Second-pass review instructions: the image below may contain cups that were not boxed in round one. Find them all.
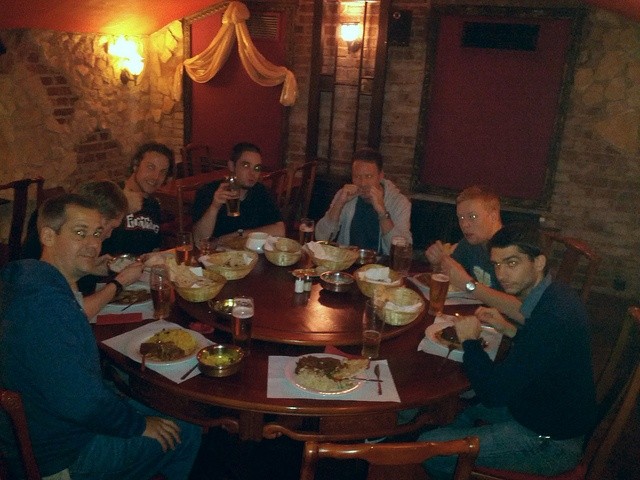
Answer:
[200,237,217,257]
[362,300,385,333]
[362,330,381,359]
[298,218,314,248]
[428,274,455,313]
[149,263,168,314]
[176,230,193,266]
[233,295,253,339]
[223,174,242,217]
[391,235,406,271]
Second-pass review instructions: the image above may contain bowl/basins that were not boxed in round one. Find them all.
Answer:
[245,233,270,253]
[208,298,233,323]
[106,254,141,277]
[355,248,377,265]
[320,270,354,293]
[198,344,245,379]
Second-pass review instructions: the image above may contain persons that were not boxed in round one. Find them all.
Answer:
[100,141,175,257]
[316,151,413,255]
[419,221,599,476]
[191,141,286,245]
[425,185,532,324]
[17,179,144,321]
[0,194,203,479]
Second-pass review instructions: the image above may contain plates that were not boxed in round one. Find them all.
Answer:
[89,280,157,307]
[285,353,366,394]
[128,327,202,366]
[293,268,321,278]
[413,271,466,292]
[425,322,496,352]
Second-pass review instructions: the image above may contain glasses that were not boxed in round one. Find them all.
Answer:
[235,160,263,170]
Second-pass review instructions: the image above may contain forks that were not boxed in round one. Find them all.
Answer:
[437,344,459,372]
[374,363,383,396]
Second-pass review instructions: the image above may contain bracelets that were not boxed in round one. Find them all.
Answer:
[109,280,124,295]
[238,228,244,234]
[379,212,390,219]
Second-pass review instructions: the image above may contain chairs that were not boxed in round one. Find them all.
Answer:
[180,143,213,178]
[0,175,45,261]
[0,392,41,480]
[473,305,640,480]
[290,161,319,225]
[300,436,481,479]
[537,230,602,304]
[178,182,202,232]
[259,170,289,220]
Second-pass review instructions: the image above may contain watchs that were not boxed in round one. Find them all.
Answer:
[465,279,478,293]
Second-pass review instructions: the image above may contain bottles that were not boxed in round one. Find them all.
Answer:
[294,275,304,294]
[304,274,312,291]
